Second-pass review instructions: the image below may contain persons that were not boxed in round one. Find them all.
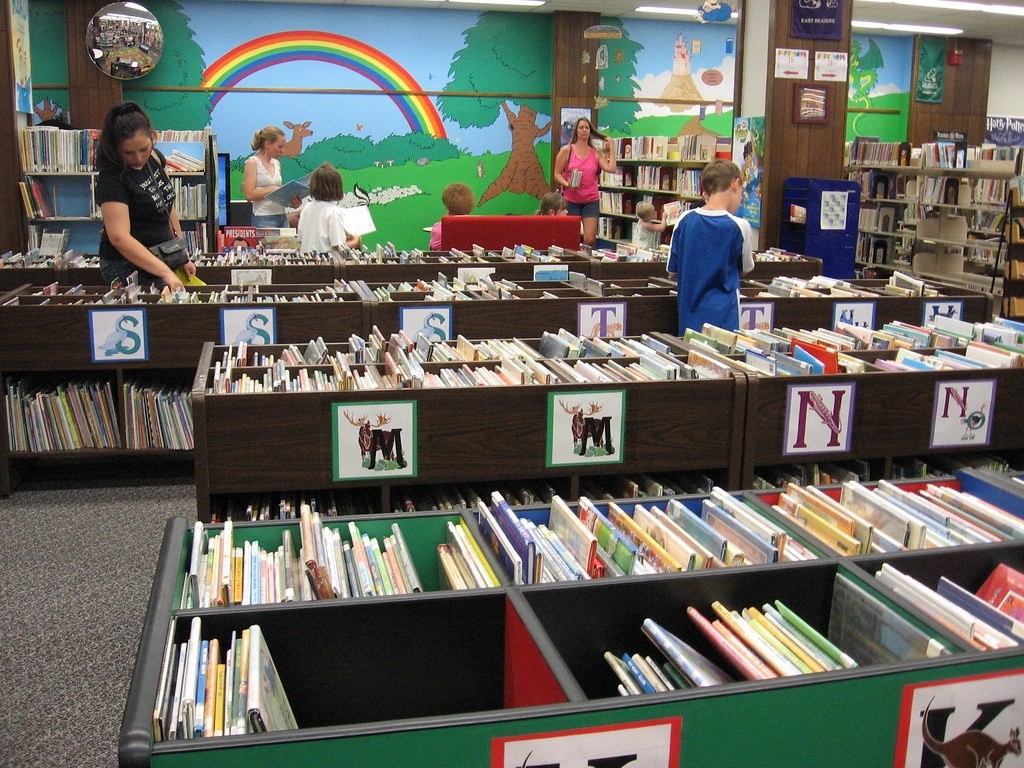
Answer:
[244,125,302,228]
[298,164,360,253]
[429,182,476,250]
[636,201,668,252]
[535,117,616,249]
[666,161,754,336]
[95,102,196,293]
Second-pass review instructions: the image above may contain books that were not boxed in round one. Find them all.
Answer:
[153,453,1024,742]
[0,126,1024,452]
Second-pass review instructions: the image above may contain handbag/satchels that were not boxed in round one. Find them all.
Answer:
[148,238,190,270]
[555,186,564,197]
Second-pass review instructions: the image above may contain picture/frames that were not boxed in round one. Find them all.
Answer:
[793,81,832,127]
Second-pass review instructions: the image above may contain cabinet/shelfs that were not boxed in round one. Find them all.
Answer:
[15,142,216,255]
[0,246,1024,768]
[839,167,1024,319]
[594,157,708,252]
